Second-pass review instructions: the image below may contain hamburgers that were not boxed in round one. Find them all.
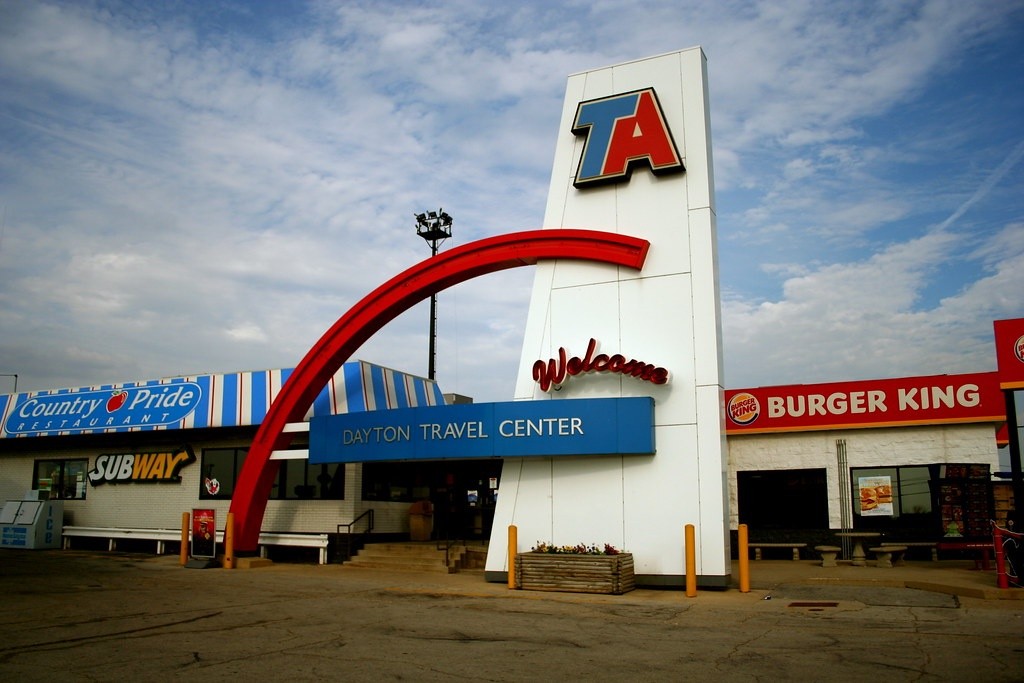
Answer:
[875,485,892,502]
[860,488,878,510]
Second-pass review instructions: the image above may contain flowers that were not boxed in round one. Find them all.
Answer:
[532,541,620,555]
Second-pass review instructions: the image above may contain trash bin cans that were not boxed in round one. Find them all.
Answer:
[409,500,432,541]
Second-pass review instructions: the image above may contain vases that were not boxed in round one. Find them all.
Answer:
[515,552,636,595]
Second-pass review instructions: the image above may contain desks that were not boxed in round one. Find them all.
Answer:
[835,532,881,566]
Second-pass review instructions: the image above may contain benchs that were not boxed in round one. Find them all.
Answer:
[870,546,910,568]
[881,543,938,563]
[748,543,807,561]
[815,545,842,567]
[937,543,995,570]
